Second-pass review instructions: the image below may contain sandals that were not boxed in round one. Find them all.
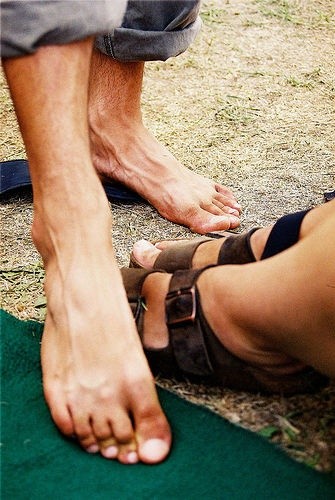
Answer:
[129,207,309,274]
[114,268,329,392]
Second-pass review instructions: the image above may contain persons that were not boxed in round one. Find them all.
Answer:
[1,0,242,464]
[120,192,334,397]
